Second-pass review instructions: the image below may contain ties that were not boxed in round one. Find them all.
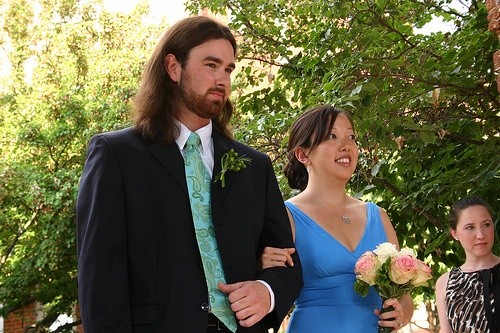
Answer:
[183,132,240,333]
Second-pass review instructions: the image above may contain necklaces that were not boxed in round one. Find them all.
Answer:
[299,191,351,223]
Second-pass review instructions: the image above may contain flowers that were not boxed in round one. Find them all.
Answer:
[354,242,434,333]
[215,148,253,188]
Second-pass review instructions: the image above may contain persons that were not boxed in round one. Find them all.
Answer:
[76,15,303,333]
[435,196,500,333]
[261,103,414,333]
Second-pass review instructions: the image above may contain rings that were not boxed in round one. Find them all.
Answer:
[398,319,402,327]
